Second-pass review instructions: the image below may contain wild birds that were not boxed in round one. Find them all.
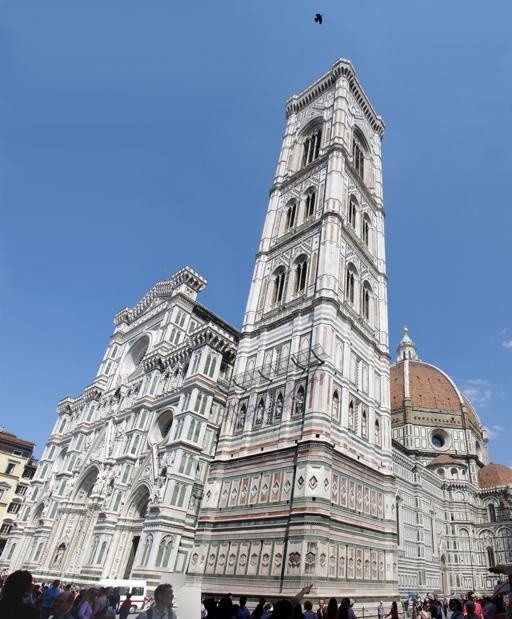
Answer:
[314,14,323,24]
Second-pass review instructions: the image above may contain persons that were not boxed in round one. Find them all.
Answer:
[384,601,398,619]
[201,584,357,619]
[0,568,132,619]
[135,583,177,619]
[402,580,512,619]
[377,600,384,619]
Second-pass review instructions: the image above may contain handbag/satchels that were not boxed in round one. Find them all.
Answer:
[71,600,88,617]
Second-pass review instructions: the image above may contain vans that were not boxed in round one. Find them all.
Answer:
[96,579,147,614]
